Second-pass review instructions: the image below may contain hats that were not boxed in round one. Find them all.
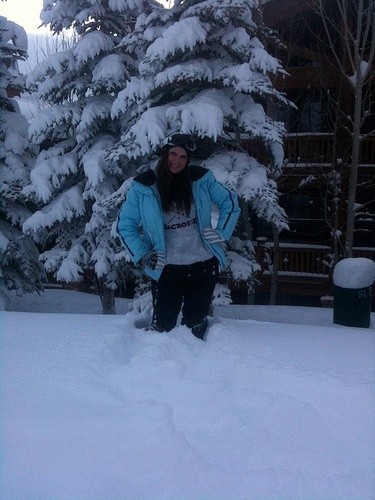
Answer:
[155,134,193,164]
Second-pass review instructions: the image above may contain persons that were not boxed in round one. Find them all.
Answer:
[115,134,240,341]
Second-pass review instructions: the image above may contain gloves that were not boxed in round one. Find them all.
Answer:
[138,251,157,270]
[202,225,225,243]
